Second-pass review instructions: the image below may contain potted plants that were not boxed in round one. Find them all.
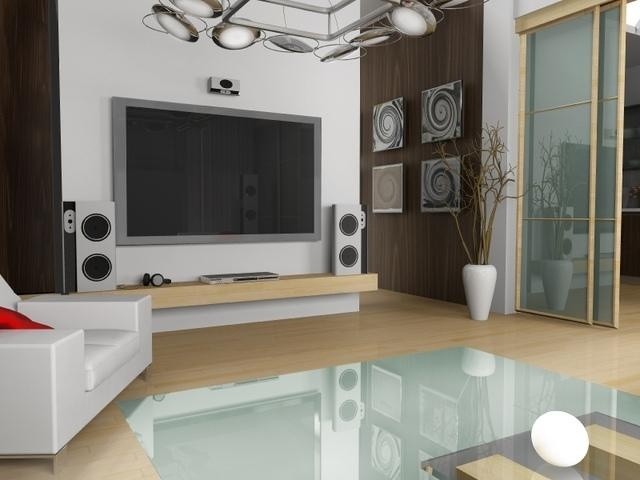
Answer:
[530,127,589,311]
[445,347,497,456]
[430,120,530,321]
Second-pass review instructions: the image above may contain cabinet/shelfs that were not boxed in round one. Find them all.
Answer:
[68,272,378,308]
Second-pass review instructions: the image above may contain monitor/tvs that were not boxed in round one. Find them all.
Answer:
[110,96,322,246]
[560,140,616,235]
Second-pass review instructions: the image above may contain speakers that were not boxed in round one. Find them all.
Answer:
[241,173,260,234]
[548,205,576,264]
[331,203,368,276]
[61,200,117,294]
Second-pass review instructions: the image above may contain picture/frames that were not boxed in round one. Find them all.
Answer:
[420,156,461,213]
[371,96,406,153]
[372,162,404,214]
[421,79,464,144]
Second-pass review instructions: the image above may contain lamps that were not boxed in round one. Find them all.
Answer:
[142,3,207,42]
[158,0,231,18]
[206,17,266,50]
[263,32,319,57]
[377,1,444,39]
[314,44,367,62]
[343,25,402,46]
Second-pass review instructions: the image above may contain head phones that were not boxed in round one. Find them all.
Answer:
[142,273,172,287]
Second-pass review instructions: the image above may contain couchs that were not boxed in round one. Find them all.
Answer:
[0,276,151,474]
